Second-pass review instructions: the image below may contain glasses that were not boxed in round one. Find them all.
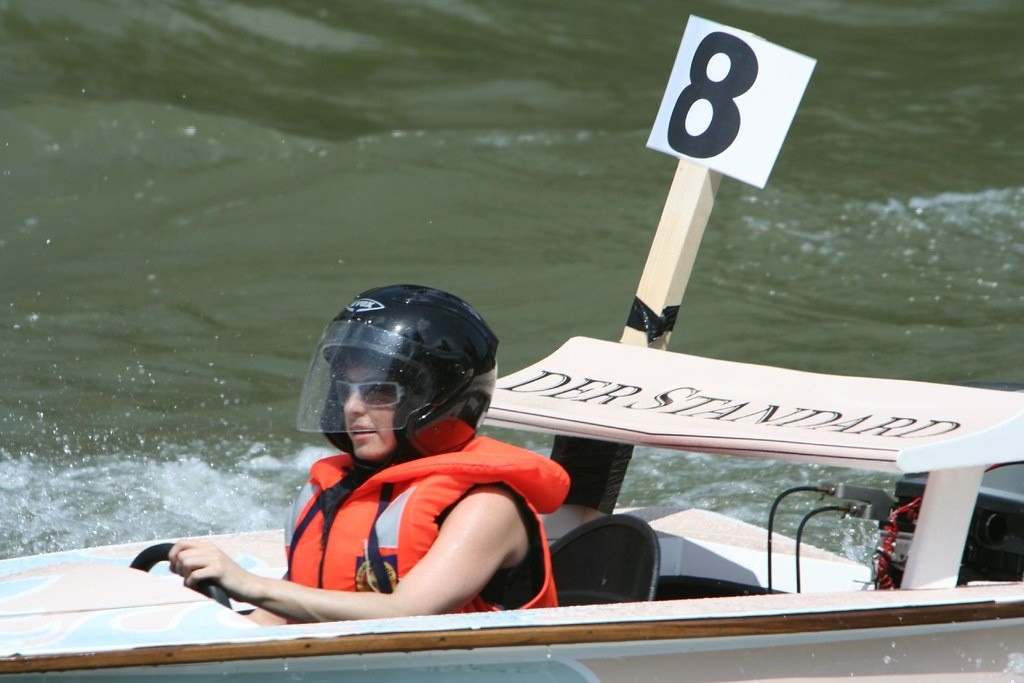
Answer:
[336,381,406,407]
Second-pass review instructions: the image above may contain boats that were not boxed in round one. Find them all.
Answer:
[0,15,1024,683]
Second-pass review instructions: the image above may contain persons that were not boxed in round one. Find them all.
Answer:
[169,285,571,627]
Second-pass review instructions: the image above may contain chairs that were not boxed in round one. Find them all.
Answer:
[549,513,661,607]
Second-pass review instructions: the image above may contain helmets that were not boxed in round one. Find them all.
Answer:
[296,284,499,453]
